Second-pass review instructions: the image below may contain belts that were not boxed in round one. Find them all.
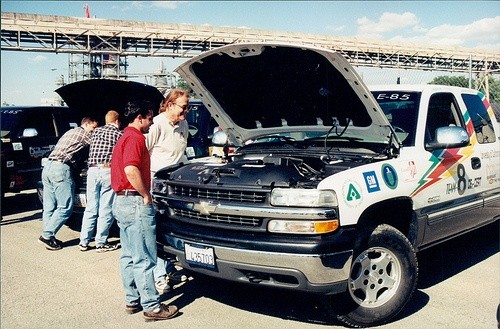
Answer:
[49,158,70,164]
[89,164,112,168]
[116,191,141,196]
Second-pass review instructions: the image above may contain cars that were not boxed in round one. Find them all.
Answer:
[37,77,219,229]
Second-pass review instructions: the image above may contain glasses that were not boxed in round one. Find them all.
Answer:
[173,103,193,112]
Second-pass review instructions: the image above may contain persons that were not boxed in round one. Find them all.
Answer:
[111,97,180,321]
[38,114,99,250]
[146,86,190,294]
[79,109,122,253]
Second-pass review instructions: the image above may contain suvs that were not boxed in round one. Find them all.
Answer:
[1,105,76,195]
[148,39,499,329]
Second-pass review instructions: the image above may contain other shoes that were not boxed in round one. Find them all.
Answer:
[96,244,116,252]
[142,304,179,321]
[154,281,172,294]
[78,244,89,251]
[38,236,62,251]
[166,270,183,283]
[125,305,143,315]
